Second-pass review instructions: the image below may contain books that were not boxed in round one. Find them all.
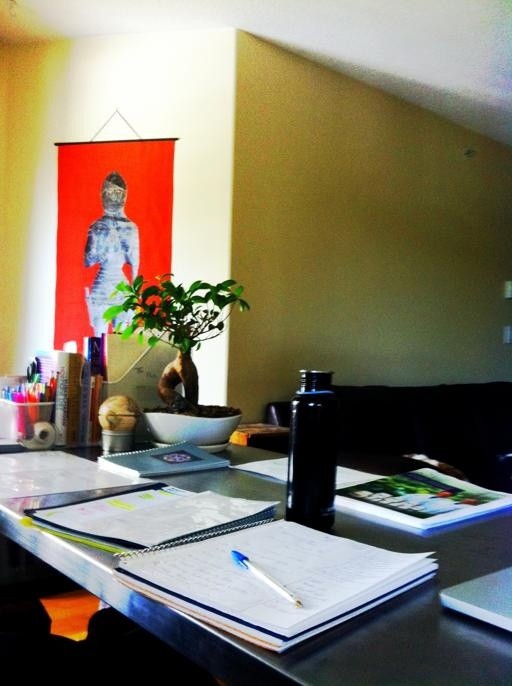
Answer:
[102,331,158,382]
[85,336,107,378]
[55,352,85,446]
[333,466,512,530]
[96,441,233,475]
[112,516,439,653]
[20,481,281,552]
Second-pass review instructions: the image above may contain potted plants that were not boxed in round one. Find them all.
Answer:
[101,271,253,454]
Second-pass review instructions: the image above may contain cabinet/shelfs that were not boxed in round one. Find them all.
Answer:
[228,423,290,455]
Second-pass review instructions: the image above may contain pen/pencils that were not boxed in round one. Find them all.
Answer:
[231,547,310,612]
[49,368,59,383]
[34,374,42,383]
[3,383,61,437]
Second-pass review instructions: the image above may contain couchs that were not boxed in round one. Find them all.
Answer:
[263,380,512,494]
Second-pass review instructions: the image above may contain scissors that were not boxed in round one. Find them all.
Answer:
[26,356,41,383]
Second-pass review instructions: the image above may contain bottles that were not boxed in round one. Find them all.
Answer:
[285,370,340,531]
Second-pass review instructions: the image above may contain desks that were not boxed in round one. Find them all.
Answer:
[0,440,511,686]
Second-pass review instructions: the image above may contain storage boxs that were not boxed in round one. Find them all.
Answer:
[0,396,56,439]
